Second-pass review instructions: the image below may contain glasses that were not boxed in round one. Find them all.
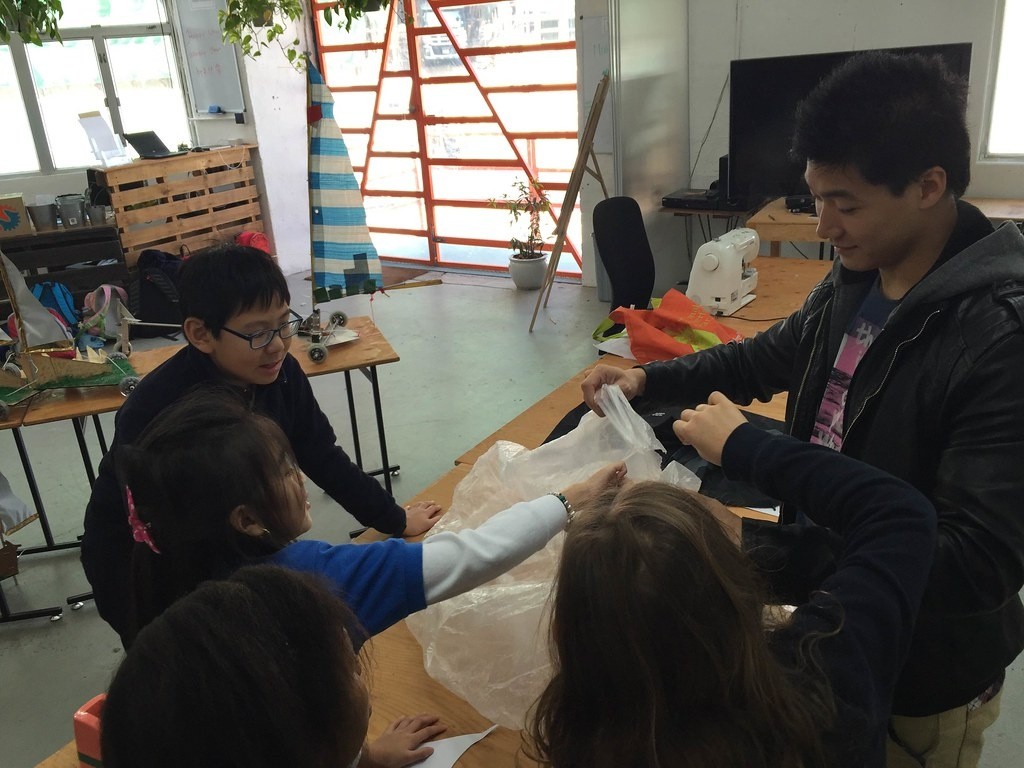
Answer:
[221,308,304,350]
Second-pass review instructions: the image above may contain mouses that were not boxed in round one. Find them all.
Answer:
[191,147,203,152]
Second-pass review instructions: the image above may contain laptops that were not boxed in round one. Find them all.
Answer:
[123,131,188,159]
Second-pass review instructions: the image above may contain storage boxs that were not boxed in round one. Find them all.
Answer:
[0,191,32,237]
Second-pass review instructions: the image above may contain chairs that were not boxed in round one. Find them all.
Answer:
[592,196,655,355]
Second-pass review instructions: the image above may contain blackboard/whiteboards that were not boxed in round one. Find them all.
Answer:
[170,0,247,114]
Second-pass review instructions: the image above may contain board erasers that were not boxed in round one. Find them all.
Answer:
[209,106,221,113]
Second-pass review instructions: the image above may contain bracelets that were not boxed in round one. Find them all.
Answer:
[546,491,574,532]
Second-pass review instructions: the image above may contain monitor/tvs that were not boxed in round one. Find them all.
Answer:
[728,43,973,203]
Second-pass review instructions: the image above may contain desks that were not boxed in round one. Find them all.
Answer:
[453,251,836,524]
[745,197,1024,260]
[0,395,64,622]
[35,462,549,768]
[22,314,401,611]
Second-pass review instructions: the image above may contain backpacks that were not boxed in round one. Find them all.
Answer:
[74,283,131,346]
[131,248,186,337]
[7,306,76,360]
[234,229,276,256]
[29,279,81,347]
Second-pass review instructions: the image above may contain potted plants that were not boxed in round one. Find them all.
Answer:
[485,176,551,291]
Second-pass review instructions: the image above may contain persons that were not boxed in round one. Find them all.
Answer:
[98,568,445,768]
[581,53,1024,768]
[113,398,628,655]
[81,243,441,658]
[521,390,939,768]
